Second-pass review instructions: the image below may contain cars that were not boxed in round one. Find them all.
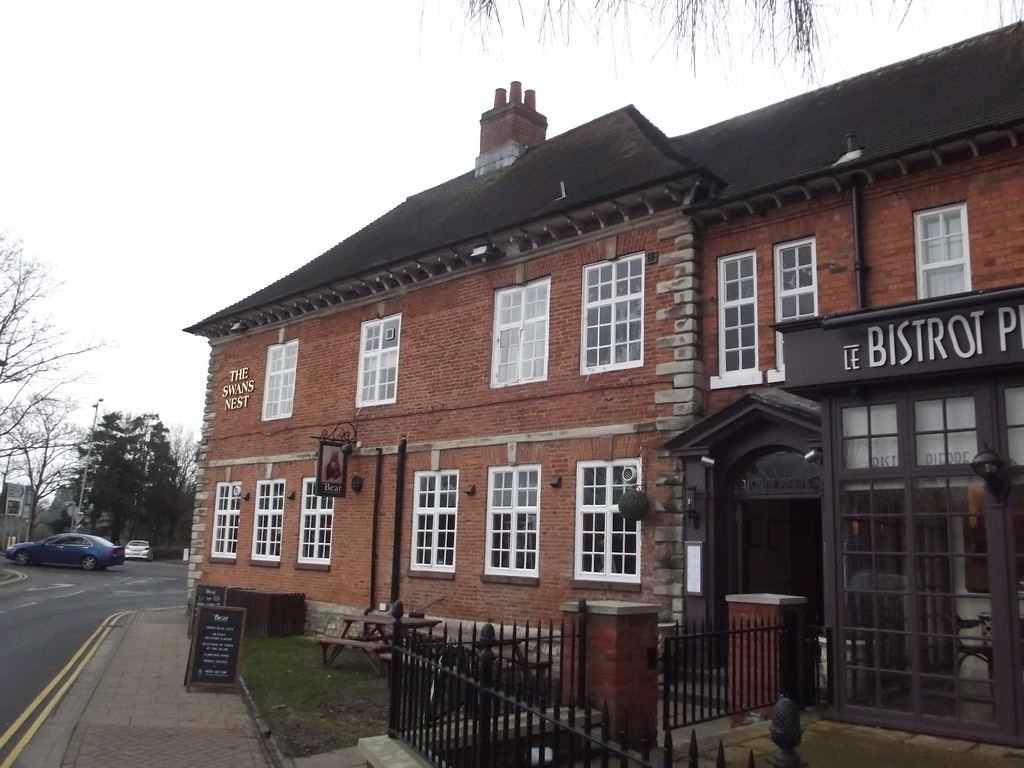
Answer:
[5,532,126,571]
[124,539,153,562]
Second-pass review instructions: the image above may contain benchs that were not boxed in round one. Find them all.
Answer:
[384,630,443,642]
[317,637,392,676]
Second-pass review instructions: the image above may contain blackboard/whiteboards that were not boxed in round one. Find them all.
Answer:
[187,605,247,685]
[180,584,228,638]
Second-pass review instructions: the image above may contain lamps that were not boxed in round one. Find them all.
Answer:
[684,484,699,531]
[967,442,1008,504]
[804,450,821,470]
[699,455,716,468]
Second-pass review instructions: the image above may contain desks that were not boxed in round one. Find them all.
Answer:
[327,615,443,676]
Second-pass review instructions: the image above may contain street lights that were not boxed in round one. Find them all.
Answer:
[74,398,105,530]
